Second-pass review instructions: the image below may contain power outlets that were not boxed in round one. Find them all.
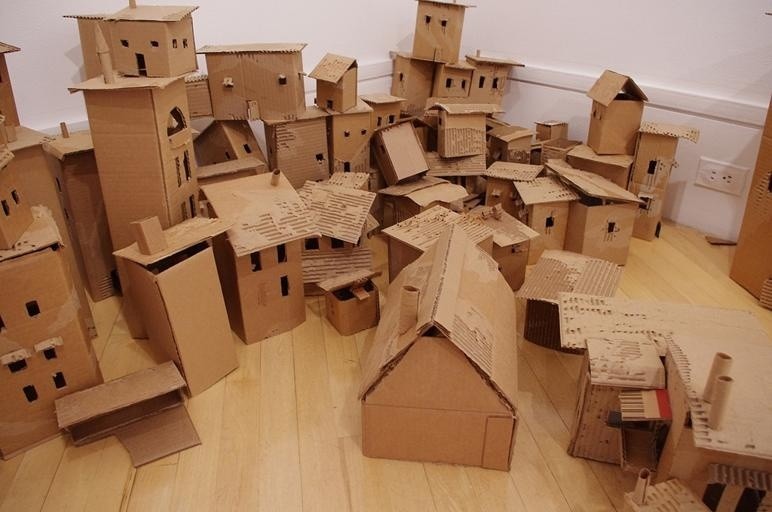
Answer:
[691,157,751,196]
